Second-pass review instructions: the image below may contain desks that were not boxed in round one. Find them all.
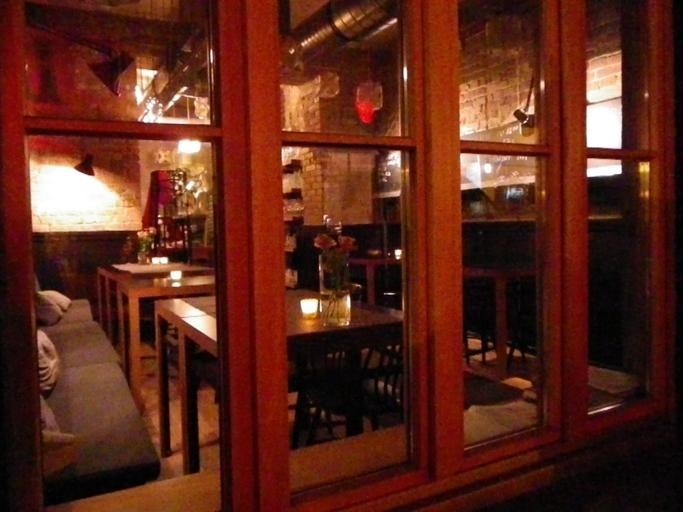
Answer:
[96,261,216,350]
[154,290,403,475]
[114,276,215,417]
[318,255,401,304]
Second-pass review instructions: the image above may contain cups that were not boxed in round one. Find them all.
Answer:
[152,257,168,265]
[299,297,319,320]
[171,270,182,282]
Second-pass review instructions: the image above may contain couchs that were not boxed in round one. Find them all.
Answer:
[33,274,161,483]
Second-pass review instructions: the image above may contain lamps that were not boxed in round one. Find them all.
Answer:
[74,154,96,176]
[513,78,533,124]
[27,21,136,97]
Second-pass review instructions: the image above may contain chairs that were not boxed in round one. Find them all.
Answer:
[294,336,404,448]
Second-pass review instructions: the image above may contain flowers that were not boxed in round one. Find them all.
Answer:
[309,234,363,326]
[136,227,157,251]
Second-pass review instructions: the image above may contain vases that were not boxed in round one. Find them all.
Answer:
[137,253,150,264]
[327,291,352,325]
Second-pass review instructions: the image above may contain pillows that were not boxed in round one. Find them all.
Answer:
[36,288,76,483]
